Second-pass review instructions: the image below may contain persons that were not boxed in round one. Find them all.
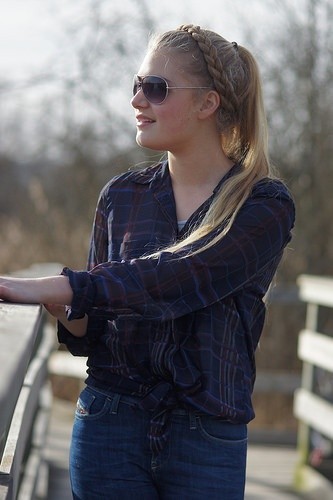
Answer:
[0,23,296,500]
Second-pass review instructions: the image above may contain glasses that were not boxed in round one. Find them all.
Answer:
[132,74,215,105]
